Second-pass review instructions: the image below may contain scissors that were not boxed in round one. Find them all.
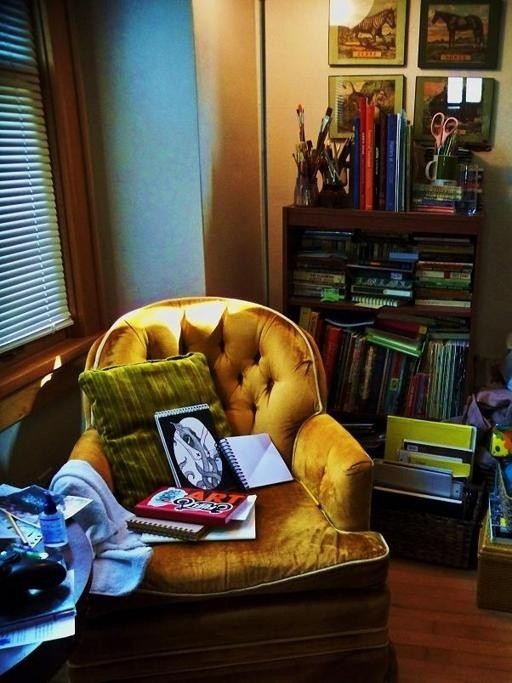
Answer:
[430,112,458,155]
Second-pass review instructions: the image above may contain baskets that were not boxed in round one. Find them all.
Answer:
[369,478,486,567]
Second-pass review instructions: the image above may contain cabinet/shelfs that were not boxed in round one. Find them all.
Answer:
[281,205,487,452]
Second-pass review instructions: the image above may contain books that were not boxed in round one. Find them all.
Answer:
[1,400,295,652]
[292,227,475,308]
[371,415,477,520]
[350,96,485,215]
[298,307,471,449]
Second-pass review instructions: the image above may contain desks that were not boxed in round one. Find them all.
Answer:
[0,518,94,683]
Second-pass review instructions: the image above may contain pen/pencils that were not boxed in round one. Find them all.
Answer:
[434,128,459,157]
[8,514,28,546]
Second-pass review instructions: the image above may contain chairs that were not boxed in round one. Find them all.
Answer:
[67,295,390,683]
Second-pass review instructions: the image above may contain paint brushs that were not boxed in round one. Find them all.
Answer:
[292,104,354,205]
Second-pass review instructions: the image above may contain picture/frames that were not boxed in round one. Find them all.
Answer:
[328,0,504,152]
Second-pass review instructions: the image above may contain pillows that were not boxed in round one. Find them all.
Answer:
[79,351,236,511]
